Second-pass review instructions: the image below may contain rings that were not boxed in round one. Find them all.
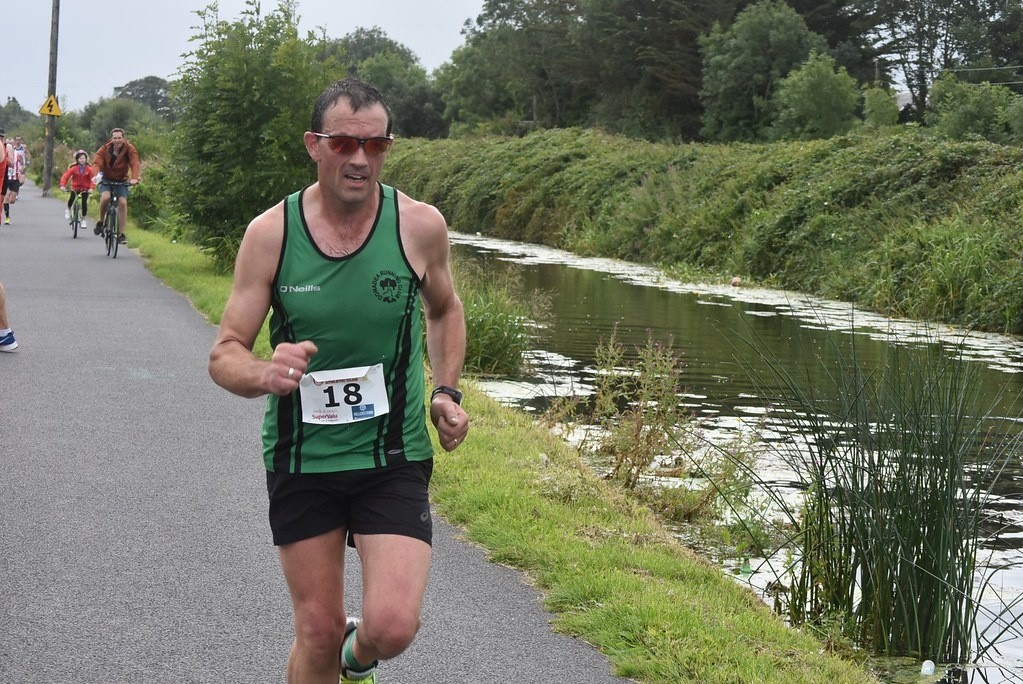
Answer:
[455,439,457,445]
[288,368,294,378]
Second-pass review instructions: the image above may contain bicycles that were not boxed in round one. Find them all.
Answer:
[61,189,92,239]
[97,180,140,260]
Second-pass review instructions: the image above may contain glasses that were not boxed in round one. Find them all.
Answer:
[314,133,394,156]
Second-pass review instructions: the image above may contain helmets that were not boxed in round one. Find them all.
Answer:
[74,150,88,159]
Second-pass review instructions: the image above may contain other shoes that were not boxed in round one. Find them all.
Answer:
[118,233,128,244]
[65,209,71,218]
[94,221,103,235]
[4,217,10,224]
[81,220,87,228]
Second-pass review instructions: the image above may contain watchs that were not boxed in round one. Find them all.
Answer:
[430,386,462,405]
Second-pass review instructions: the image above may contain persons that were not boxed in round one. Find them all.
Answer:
[60,150,95,229]
[0,282,18,351]
[92,128,139,245]
[0,129,31,226]
[209,78,469,684]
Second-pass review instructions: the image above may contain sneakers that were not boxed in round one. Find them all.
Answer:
[340,617,378,684]
[0,331,18,352]
